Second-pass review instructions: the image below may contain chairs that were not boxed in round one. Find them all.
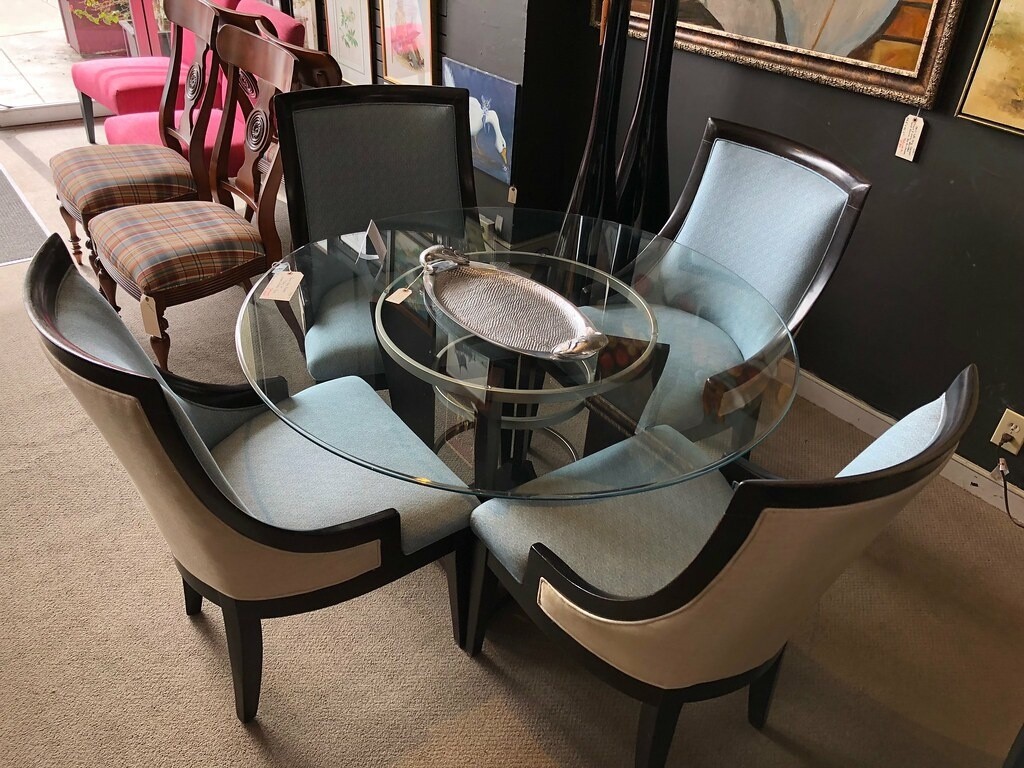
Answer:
[47,0,980,768]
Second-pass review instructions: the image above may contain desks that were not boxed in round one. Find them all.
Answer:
[234,206,797,502]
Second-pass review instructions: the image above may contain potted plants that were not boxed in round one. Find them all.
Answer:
[69,0,172,56]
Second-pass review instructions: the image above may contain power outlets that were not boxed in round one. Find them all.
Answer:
[991,407,1024,455]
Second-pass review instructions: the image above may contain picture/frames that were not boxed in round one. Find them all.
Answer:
[379,0,436,86]
[595,1,963,106]
[289,0,320,51]
[957,0,1024,138]
[324,0,375,85]
